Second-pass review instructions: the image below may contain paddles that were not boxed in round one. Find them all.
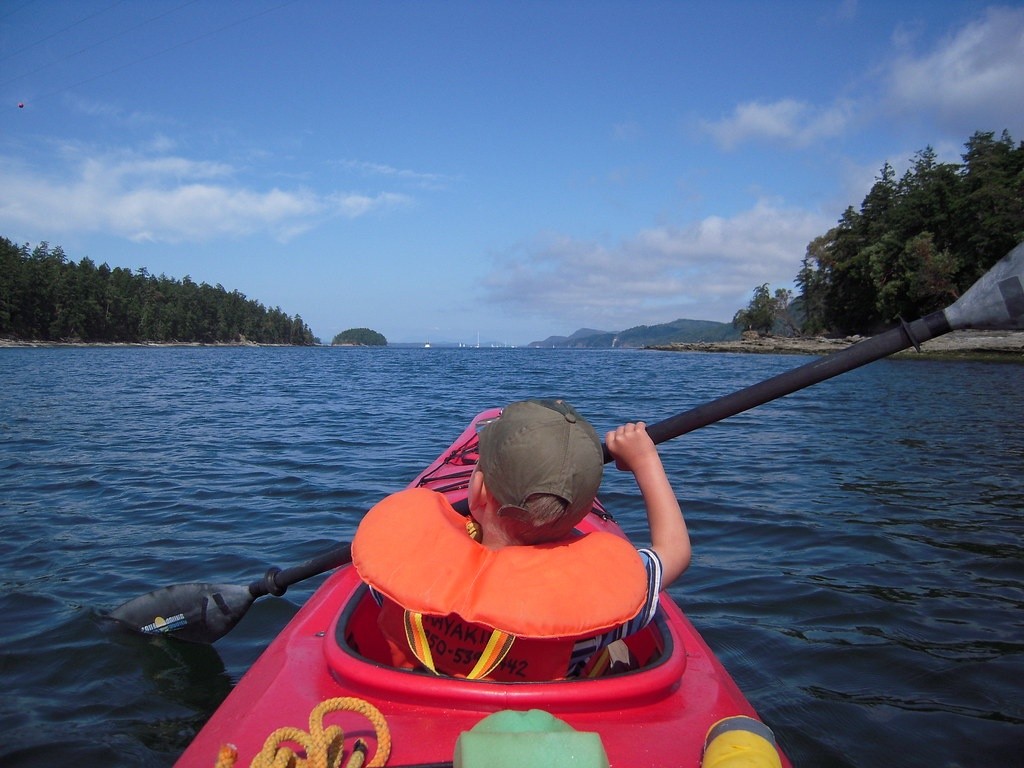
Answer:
[109,239,1024,644]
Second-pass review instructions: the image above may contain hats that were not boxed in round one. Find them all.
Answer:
[475,400,604,530]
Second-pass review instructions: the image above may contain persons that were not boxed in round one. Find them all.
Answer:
[351,396,691,688]
